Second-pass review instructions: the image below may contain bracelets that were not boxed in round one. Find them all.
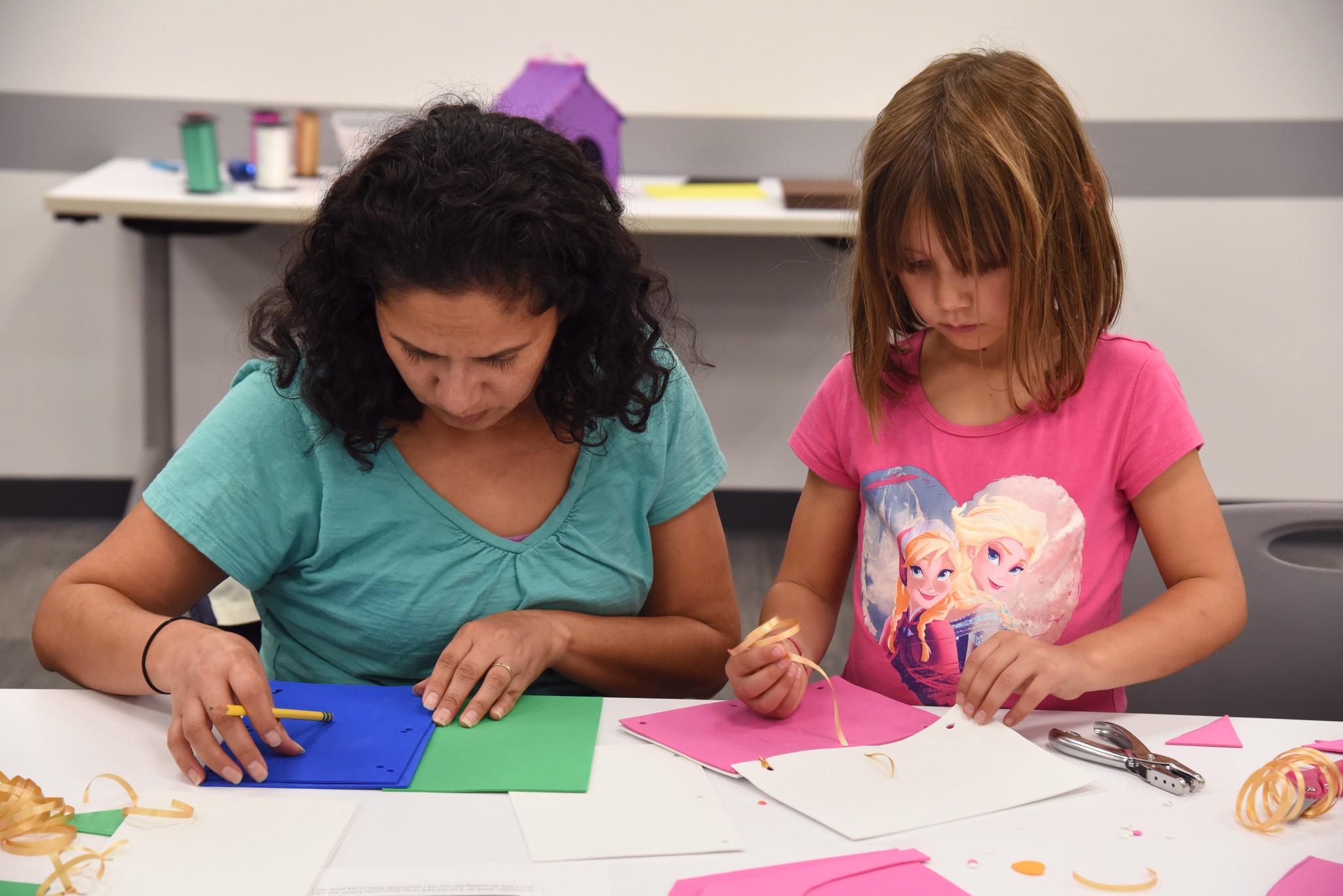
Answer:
[772,634,811,677]
[143,616,190,696]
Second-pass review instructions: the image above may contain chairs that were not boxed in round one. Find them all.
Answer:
[1119,498,1343,720]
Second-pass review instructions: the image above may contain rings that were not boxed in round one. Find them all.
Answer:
[490,663,514,683]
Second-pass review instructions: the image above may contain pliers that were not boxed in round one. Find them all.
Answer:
[1050,720,1205,796]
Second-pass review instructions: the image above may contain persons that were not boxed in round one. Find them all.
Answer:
[724,51,1247,730]
[35,90,743,786]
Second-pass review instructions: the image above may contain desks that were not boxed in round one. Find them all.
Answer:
[40,155,863,518]
[0,683,1343,895]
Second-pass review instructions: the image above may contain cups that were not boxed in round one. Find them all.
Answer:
[249,108,276,165]
[257,122,298,192]
[298,108,318,178]
[179,110,218,192]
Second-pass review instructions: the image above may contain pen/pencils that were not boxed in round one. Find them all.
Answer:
[209,704,335,721]
[148,158,181,171]
